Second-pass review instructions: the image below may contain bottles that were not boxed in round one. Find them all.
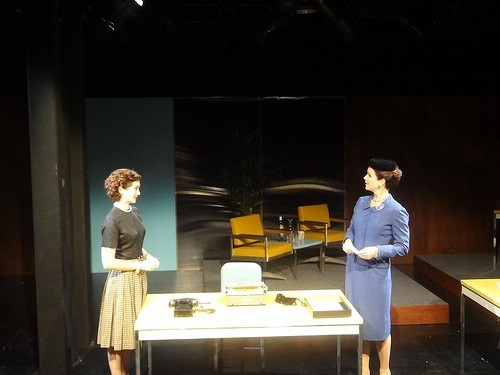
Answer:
[286,219,296,244]
[278,215,285,240]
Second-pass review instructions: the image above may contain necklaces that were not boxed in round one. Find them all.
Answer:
[373,199,384,202]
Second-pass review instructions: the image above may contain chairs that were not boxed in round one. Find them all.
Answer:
[229,214,294,275]
[298,204,348,266]
[213,262,267,373]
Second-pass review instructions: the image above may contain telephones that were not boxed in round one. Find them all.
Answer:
[169,298,198,316]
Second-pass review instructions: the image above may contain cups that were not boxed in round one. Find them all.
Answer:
[298,231,305,240]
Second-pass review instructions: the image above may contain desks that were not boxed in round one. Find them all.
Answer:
[134,288,364,375]
[459,278,500,375]
[268,237,324,280]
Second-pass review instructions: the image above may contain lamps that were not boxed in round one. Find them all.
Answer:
[293,0,324,15]
[97,0,145,33]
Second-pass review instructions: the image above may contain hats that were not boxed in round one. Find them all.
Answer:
[369,159,396,171]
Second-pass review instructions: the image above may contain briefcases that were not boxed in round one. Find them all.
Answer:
[225,282,268,305]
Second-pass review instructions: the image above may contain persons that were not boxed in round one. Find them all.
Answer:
[342,158,410,375]
[97,168,160,375]
